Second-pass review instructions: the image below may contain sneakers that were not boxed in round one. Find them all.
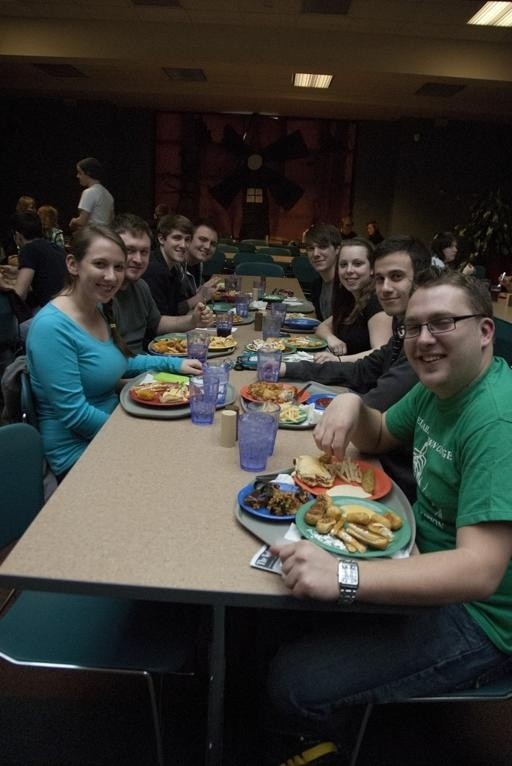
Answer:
[261,736,339,765]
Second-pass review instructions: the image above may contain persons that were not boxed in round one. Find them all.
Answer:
[257,234,432,507]
[184,218,222,291]
[305,224,343,324]
[140,213,221,316]
[313,237,394,369]
[336,214,360,242]
[25,222,204,485]
[109,213,214,356]
[37,205,65,248]
[430,230,458,272]
[68,157,115,230]
[233,265,512,766]
[10,208,69,316]
[497,272,512,294]
[366,220,384,248]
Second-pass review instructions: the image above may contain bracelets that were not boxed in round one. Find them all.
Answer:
[336,354,344,364]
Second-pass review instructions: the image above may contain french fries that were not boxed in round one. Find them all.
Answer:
[326,454,363,484]
[338,529,367,554]
[138,382,165,393]
[333,518,346,535]
[165,345,177,353]
[278,403,302,422]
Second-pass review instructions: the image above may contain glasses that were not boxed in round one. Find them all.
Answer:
[394,314,487,340]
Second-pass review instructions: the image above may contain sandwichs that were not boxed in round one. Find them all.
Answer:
[295,455,334,488]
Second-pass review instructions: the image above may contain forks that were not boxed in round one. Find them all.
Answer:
[494,271,506,289]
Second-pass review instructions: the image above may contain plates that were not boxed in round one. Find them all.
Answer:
[148,332,236,357]
[229,302,258,310]
[119,370,237,418]
[278,336,328,349]
[234,466,416,557]
[240,382,312,405]
[208,337,238,349]
[245,343,297,354]
[128,382,200,407]
[206,302,234,313]
[238,482,315,519]
[295,496,411,557]
[280,328,316,333]
[294,460,392,499]
[307,394,337,410]
[240,377,317,429]
[267,302,315,313]
[283,319,321,329]
[214,314,255,325]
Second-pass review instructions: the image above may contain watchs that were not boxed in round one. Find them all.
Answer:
[337,556,363,610]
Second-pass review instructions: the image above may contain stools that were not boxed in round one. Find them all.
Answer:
[349,676,512,766]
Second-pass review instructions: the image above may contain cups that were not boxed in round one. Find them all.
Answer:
[231,276,242,291]
[490,285,500,302]
[235,293,250,318]
[199,287,217,310]
[271,302,287,328]
[479,278,491,291]
[257,348,282,384]
[225,278,237,296]
[186,330,211,364]
[262,316,282,341]
[247,400,281,456]
[215,311,233,337]
[237,412,275,472]
[203,365,229,404]
[188,375,220,425]
[253,279,265,301]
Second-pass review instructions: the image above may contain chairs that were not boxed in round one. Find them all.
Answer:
[0,424,196,766]
[233,252,274,270]
[218,239,299,256]
[203,251,225,275]
[292,256,320,283]
[235,261,283,278]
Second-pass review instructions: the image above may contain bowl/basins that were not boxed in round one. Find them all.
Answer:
[262,294,284,305]
